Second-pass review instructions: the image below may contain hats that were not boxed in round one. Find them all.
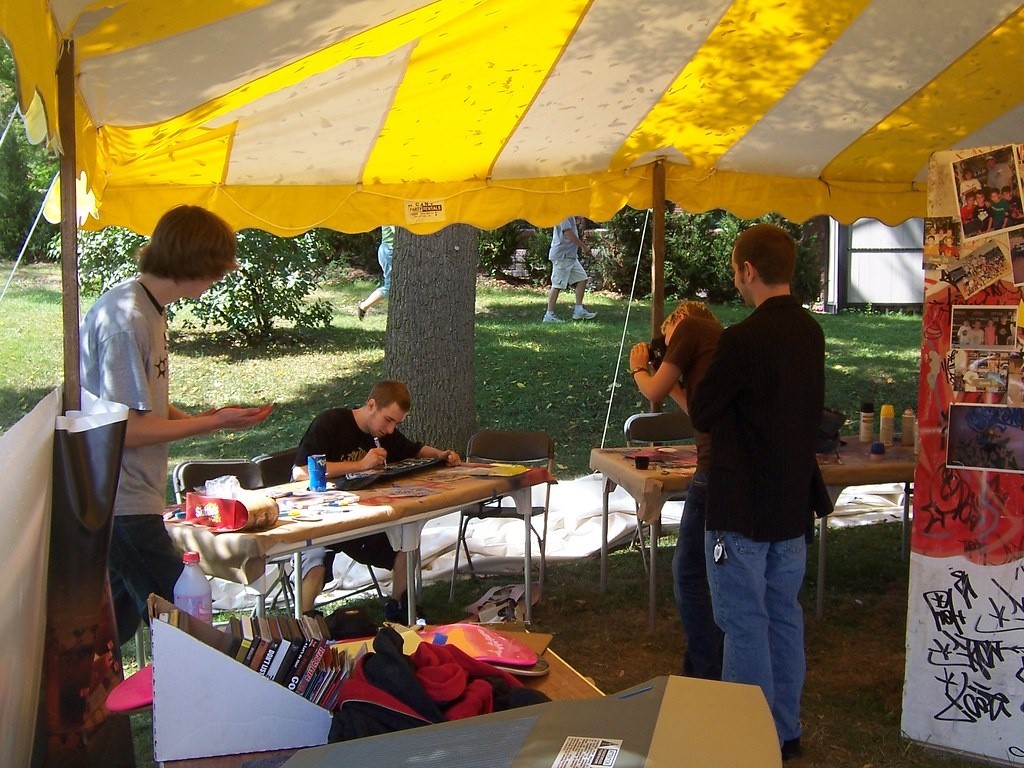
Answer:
[986,156,994,161]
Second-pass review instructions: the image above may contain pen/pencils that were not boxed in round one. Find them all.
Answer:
[272,491,293,499]
[373,437,387,466]
[621,686,653,699]
[316,509,352,515]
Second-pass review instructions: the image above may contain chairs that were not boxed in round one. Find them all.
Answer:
[170,460,294,622]
[449,431,554,607]
[249,448,383,620]
[622,413,698,574]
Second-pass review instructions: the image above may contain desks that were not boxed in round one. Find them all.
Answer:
[162,465,559,629]
[161,624,607,768]
[589,433,915,631]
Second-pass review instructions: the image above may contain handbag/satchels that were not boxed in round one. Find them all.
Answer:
[816,406,848,465]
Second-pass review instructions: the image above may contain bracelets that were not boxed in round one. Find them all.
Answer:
[631,367,648,379]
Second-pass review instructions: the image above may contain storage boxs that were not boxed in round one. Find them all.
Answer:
[145,592,336,762]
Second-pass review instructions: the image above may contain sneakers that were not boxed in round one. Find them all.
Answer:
[542,313,565,323]
[572,308,598,319]
[384,589,432,626]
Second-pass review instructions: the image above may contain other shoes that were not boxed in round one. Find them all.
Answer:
[779,736,800,760]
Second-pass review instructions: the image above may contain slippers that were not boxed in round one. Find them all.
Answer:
[357,299,366,321]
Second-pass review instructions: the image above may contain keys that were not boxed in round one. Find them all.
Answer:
[713,543,726,564]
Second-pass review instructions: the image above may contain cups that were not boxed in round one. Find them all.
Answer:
[307,454,328,491]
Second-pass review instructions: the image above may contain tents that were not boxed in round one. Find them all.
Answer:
[1,0,1024,537]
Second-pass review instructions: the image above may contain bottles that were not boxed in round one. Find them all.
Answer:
[879,404,895,447]
[173,551,213,626]
[858,399,874,442]
[901,404,916,446]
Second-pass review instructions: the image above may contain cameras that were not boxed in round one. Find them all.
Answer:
[647,336,669,370]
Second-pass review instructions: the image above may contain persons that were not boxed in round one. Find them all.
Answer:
[80,204,274,640]
[923,226,959,258]
[542,216,597,323]
[358,225,396,322]
[960,155,1024,237]
[688,225,834,759]
[290,381,461,630]
[961,252,1009,295]
[957,314,1011,345]
[629,300,724,681]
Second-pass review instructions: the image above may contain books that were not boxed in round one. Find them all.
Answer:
[157,609,351,712]
